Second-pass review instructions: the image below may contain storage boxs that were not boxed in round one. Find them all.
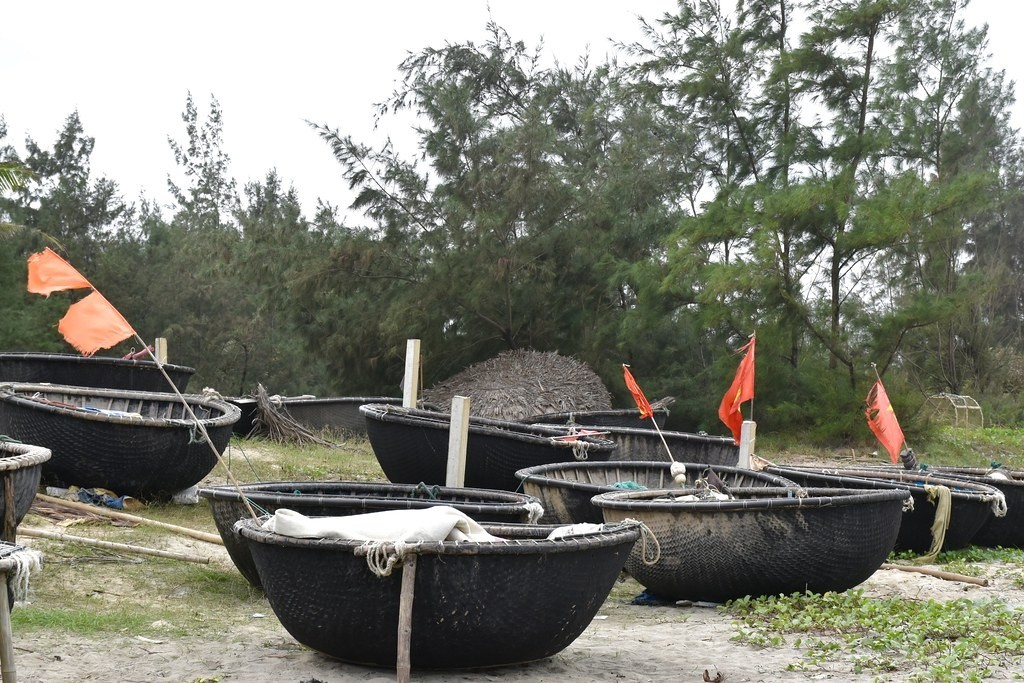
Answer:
[531,423,743,468]
[503,409,673,430]
[230,510,647,670]
[359,404,619,496]
[1,382,243,502]
[764,447,1024,555]
[0,351,197,394]
[0,440,52,538]
[590,487,911,598]
[270,394,403,442]
[194,479,546,592]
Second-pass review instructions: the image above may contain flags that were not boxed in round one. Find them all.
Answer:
[717,337,754,447]
[560,427,601,441]
[863,381,904,464]
[26,247,92,297]
[57,291,133,360]
[622,368,654,420]
